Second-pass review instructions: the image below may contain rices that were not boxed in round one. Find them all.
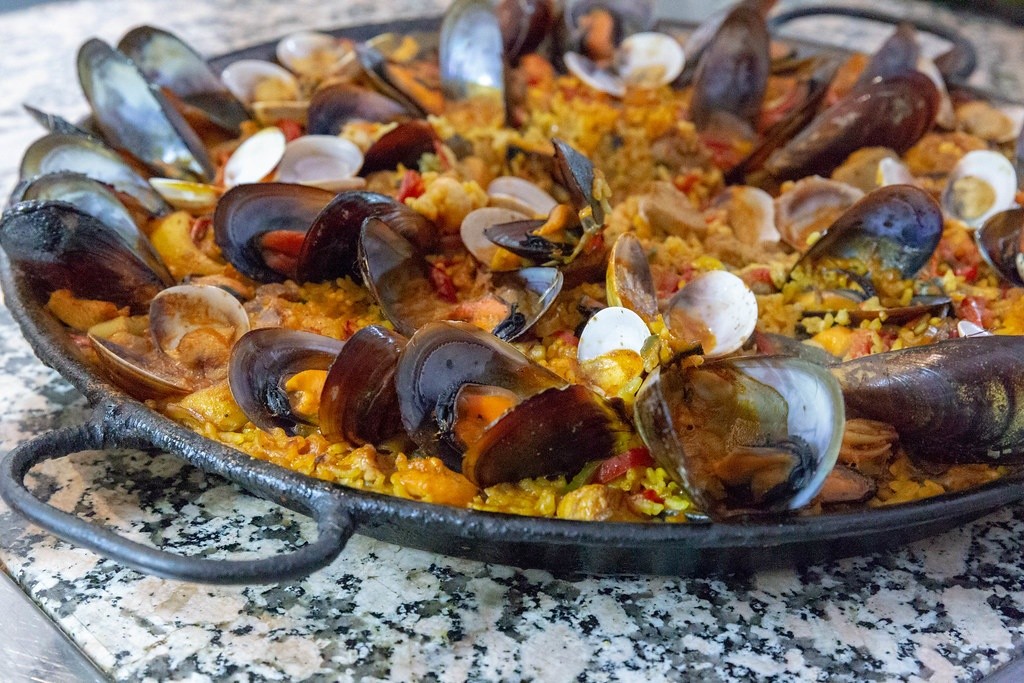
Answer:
[94,68,1024,524]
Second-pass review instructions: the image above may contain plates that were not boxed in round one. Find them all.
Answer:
[0,1,1024,582]
